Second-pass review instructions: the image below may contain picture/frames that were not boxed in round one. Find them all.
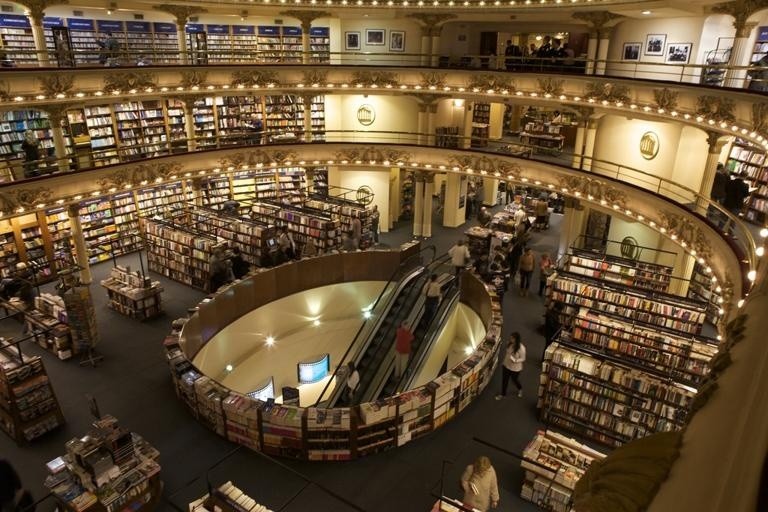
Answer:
[644,33,666,56]
[345,31,361,50]
[365,28,385,46]
[621,41,643,63]
[665,43,693,65]
[388,30,406,52]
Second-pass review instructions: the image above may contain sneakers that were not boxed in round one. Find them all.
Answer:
[495,395,506,400]
[731,235,739,240]
[520,288,529,298]
[518,389,523,398]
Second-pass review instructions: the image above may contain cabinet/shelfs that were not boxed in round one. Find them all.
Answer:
[538,304,723,448]
[189,480,273,512]
[430,496,479,512]
[722,138,766,229]
[546,232,708,339]
[0,15,330,71]
[0,169,380,358]
[520,429,608,511]
[168,242,504,462]
[43,413,160,512]
[0,337,64,446]
[1,93,326,183]
[465,190,584,283]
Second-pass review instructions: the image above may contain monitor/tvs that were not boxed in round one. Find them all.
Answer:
[46,456,65,475]
[266,237,277,249]
[71,123,84,137]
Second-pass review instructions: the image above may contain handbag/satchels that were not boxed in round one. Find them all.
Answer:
[514,271,521,288]
